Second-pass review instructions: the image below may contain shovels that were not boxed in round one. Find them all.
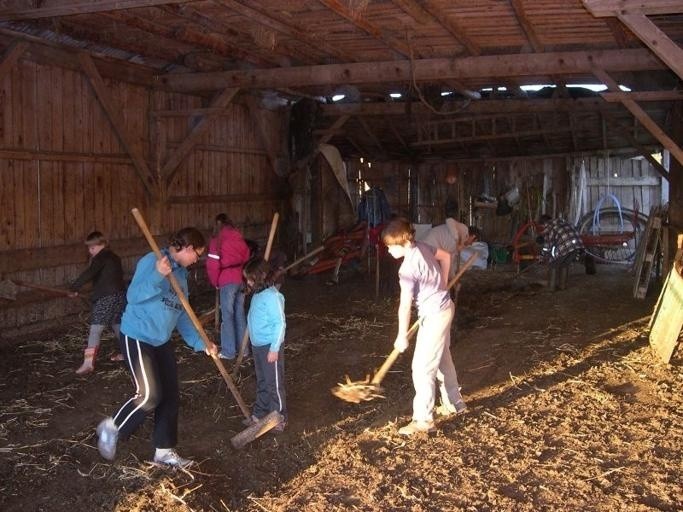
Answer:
[330,250,480,404]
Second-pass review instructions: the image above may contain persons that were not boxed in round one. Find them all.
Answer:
[422,216,481,293]
[380,215,468,435]
[95,226,219,468]
[66,230,125,376]
[240,255,288,433]
[205,212,254,361]
[537,213,583,293]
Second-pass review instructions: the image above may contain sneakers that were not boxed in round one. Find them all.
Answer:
[153,448,194,472]
[94,417,119,461]
[436,402,466,416]
[397,418,436,437]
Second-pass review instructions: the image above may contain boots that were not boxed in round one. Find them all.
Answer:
[109,351,126,364]
[75,346,97,375]
[540,265,569,294]
